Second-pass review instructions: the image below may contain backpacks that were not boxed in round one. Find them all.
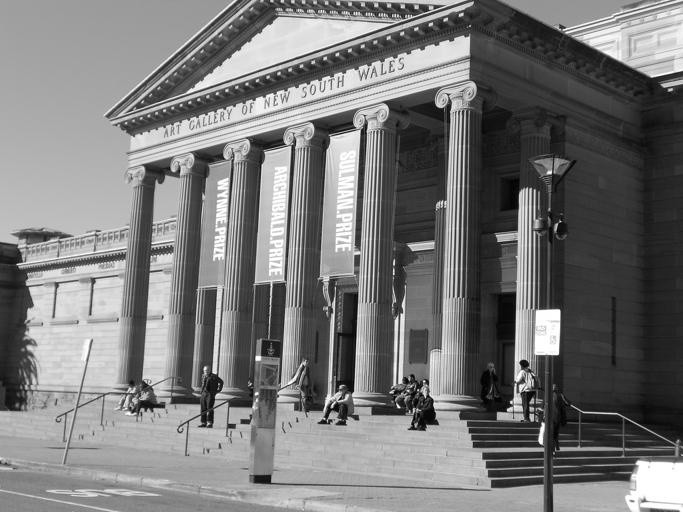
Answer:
[525,369,539,390]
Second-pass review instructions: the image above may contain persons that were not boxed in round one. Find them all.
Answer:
[115,381,137,410]
[392,374,438,432]
[317,384,354,425]
[289,358,317,412]
[512,359,539,422]
[128,383,157,415]
[198,366,223,428]
[479,362,502,406]
[540,384,566,455]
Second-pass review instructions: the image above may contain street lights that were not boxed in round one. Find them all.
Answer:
[528,151,574,512]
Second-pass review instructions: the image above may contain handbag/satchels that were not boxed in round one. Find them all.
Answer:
[538,421,546,446]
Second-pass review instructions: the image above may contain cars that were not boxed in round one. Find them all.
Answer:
[624,455,683,512]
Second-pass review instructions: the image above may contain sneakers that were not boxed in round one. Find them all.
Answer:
[335,420,346,425]
[114,406,131,411]
[198,423,213,428]
[317,419,327,424]
[408,425,425,431]
[125,411,139,416]
